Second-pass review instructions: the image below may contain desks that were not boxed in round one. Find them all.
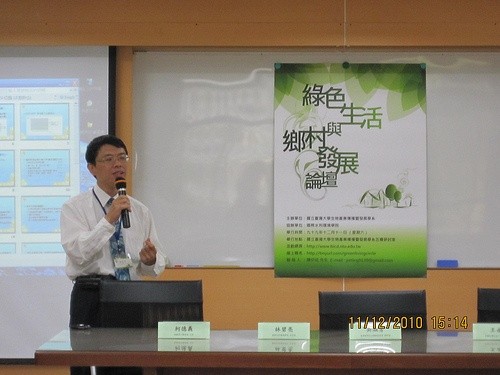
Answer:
[35,327,500,375]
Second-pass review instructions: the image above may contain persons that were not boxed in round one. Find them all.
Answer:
[58,136,167,375]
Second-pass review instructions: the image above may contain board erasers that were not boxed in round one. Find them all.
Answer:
[435,259,458,267]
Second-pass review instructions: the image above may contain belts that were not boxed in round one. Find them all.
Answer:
[76,278,103,285]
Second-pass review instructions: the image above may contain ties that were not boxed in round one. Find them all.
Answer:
[107,198,132,282]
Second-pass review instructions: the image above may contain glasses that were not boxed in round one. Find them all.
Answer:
[94,155,130,165]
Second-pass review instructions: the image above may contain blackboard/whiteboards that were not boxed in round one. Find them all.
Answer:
[130,51,500,270]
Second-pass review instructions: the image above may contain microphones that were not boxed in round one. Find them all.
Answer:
[115,177,130,227]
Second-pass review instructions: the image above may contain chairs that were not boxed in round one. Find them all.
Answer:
[78,280,203,375]
[477,287,500,323]
[318,289,428,331]
